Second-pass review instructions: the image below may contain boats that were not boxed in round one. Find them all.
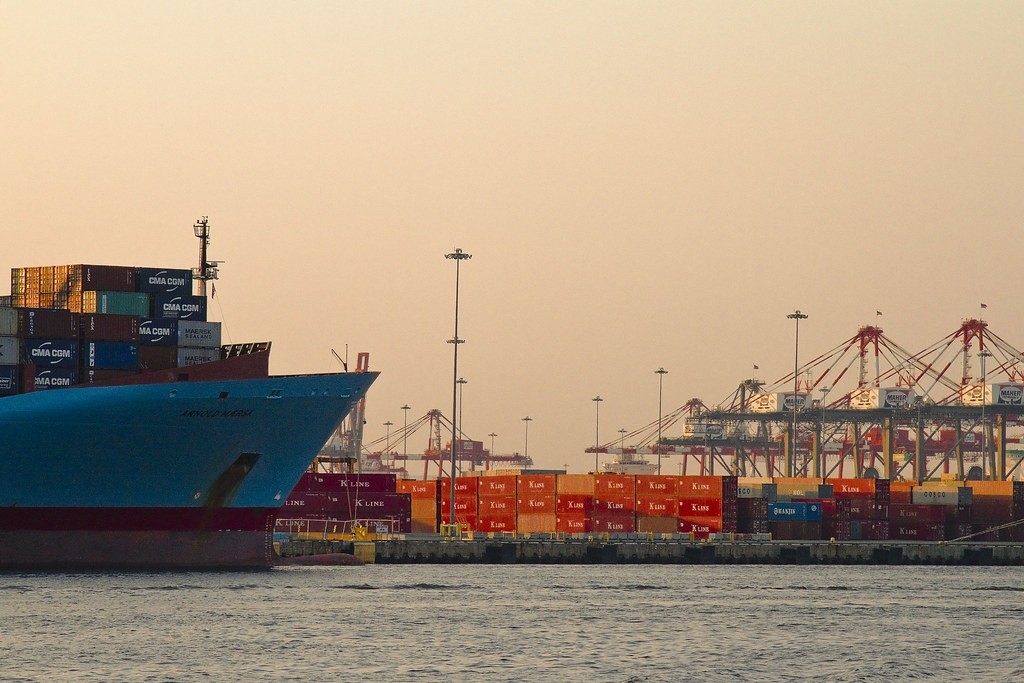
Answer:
[0,265,381,571]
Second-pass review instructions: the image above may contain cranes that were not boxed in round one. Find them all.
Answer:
[312,348,537,476]
[583,305,1022,486]
[185,216,221,311]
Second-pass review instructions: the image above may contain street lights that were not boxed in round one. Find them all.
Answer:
[443,244,473,526]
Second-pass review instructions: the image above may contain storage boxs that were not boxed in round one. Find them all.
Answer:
[275,469,1024,563]
[0,264,222,398]
